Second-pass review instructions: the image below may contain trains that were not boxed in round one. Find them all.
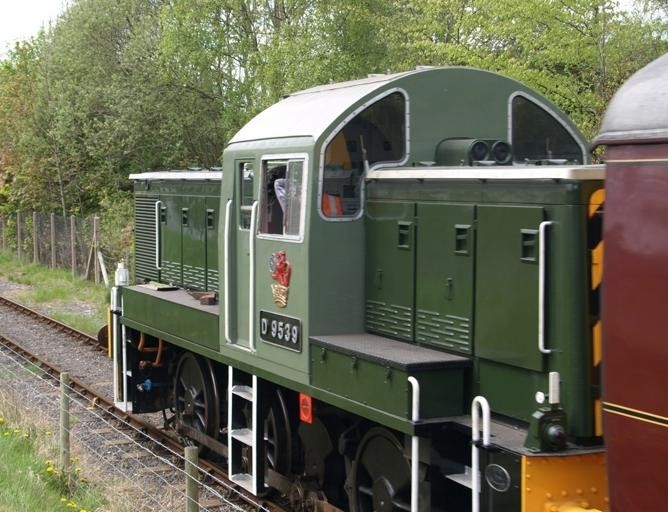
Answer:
[98,52,668,512]
